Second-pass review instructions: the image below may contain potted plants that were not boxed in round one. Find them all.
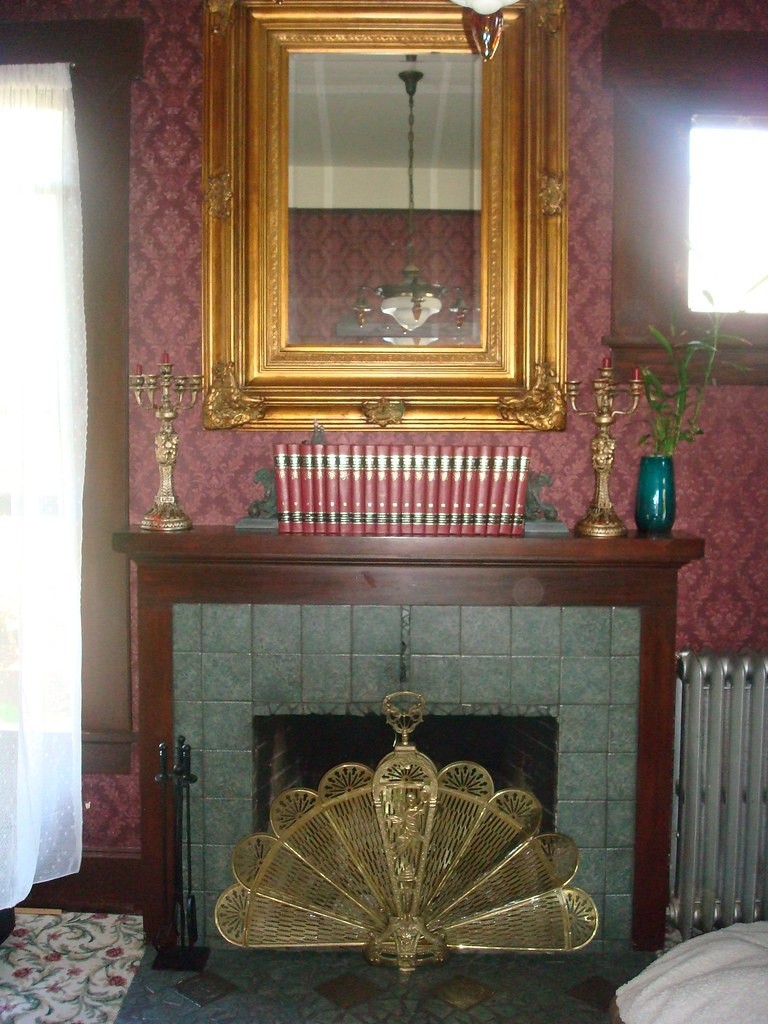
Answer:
[633,289,753,537]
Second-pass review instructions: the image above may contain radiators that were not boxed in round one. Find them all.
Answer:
[660,645,767,946]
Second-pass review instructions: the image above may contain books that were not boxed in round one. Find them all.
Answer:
[270,443,530,535]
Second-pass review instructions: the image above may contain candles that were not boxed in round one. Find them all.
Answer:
[162,351,170,364]
[631,363,640,380]
[134,361,142,376]
[602,354,611,367]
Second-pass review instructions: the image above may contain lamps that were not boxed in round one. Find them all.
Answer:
[352,56,469,332]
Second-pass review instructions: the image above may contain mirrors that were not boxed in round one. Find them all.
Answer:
[200,1,570,438]
